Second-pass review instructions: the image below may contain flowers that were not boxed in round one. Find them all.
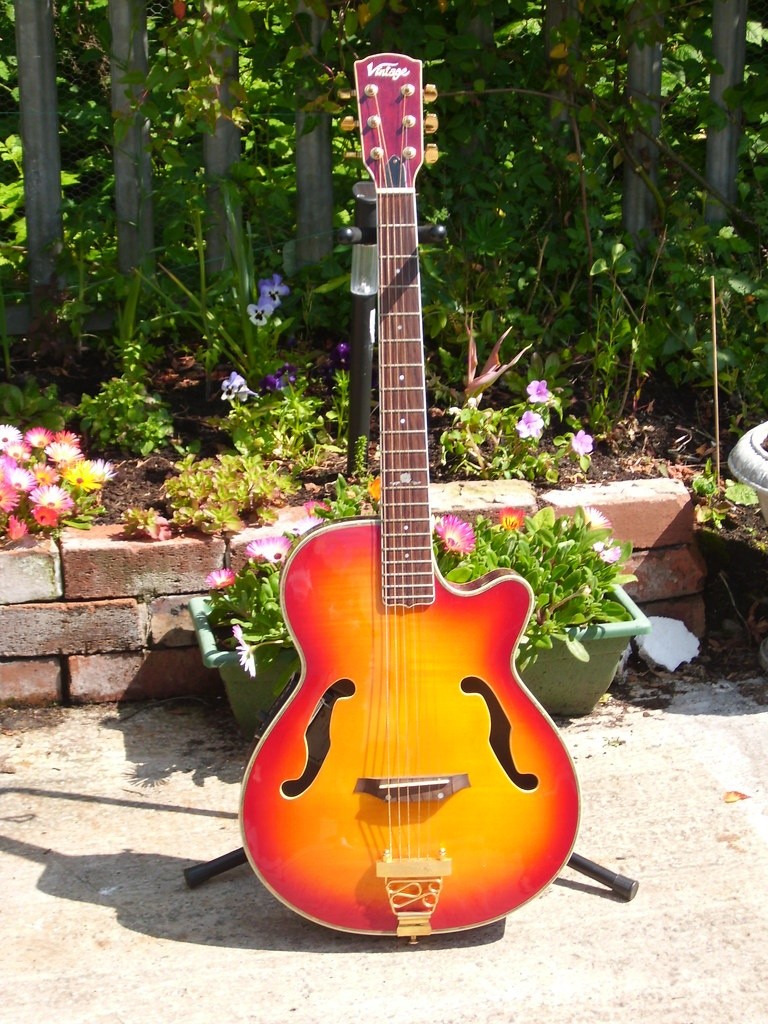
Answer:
[202,505,638,678]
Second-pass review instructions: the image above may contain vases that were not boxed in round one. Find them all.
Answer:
[188,585,652,727]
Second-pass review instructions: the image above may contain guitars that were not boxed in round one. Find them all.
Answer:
[236,51,583,941]
